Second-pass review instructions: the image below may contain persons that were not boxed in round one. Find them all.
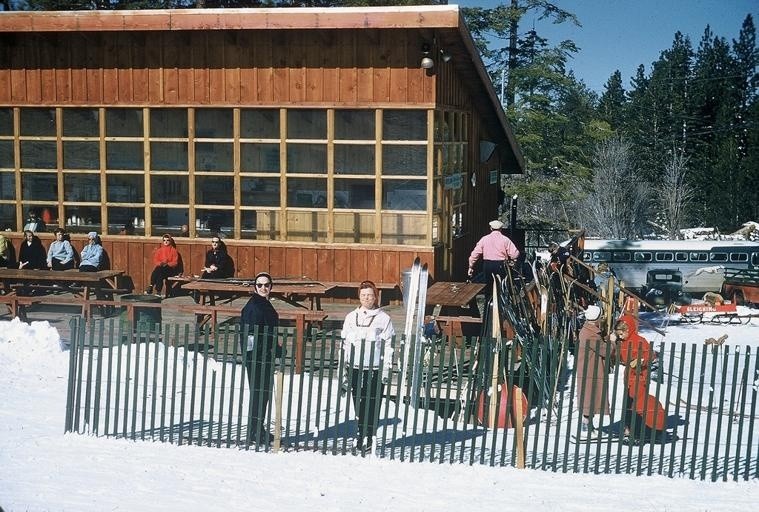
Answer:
[609,315,657,435]
[534,255,621,295]
[69,232,106,290]
[468,220,520,290]
[189,237,229,304]
[23,198,229,238]
[1,232,15,295]
[625,382,665,443]
[337,281,397,450]
[571,305,616,438]
[143,234,181,298]
[19,230,46,294]
[239,273,287,444]
[48,227,75,294]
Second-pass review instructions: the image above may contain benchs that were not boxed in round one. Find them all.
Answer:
[0,260,398,352]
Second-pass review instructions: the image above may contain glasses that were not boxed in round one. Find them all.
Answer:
[212,241,218,243]
[256,283,270,288]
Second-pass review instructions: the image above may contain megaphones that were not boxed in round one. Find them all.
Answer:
[419,39,433,55]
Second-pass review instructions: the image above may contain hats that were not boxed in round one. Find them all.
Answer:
[489,220,503,229]
[584,305,602,323]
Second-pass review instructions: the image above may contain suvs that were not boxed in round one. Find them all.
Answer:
[638,267,692,313]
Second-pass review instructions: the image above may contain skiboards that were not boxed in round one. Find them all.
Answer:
[569,434,691,444]
[182,436,359,452]
[395,257,429,429]
[496,247,665,419]
[668,399,758,419]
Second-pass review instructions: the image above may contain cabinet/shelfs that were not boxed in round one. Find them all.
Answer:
[255,209,429,248]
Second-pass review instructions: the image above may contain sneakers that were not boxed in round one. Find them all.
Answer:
[69,283,80,287]
[578,429,598,440]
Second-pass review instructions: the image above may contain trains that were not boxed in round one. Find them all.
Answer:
[552,237,759,301]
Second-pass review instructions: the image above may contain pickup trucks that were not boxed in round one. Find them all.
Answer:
[721,266,759,306]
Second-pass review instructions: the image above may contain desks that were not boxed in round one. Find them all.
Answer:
[416,281,487,350]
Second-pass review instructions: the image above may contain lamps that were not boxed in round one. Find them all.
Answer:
[440,46,452,62]
[420,42,434,69]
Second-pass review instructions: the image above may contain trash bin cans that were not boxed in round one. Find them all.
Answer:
[120,295,161,344]
[402,267,430,307]
